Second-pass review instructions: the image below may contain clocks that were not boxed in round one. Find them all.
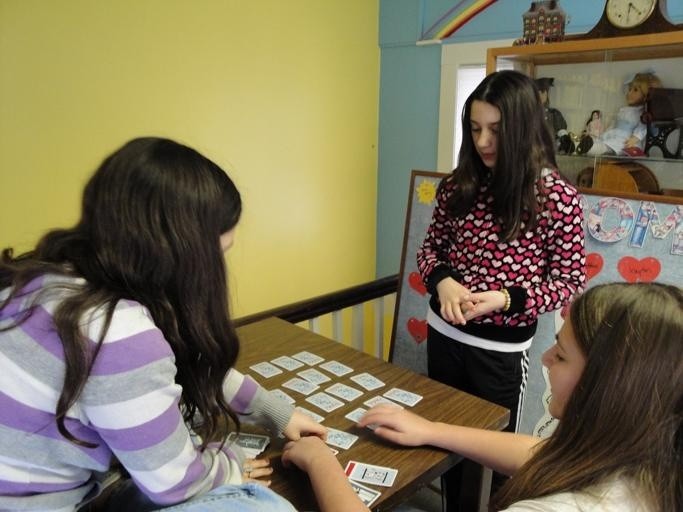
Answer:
[584,0,681,39]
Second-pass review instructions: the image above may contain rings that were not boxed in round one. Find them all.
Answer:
[242,465,252,478]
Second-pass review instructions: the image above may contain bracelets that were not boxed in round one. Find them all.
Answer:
[499,288,512,314]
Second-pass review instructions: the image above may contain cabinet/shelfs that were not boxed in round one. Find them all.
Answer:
[480,29,682,207]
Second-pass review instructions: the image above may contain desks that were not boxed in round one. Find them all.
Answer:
[173,315,514,512]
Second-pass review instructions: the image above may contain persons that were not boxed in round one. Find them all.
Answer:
[416,70,589,511]
[575,69,660,156]
[0,134,327,511]
[534,77,577,153]
[280,282,683,512]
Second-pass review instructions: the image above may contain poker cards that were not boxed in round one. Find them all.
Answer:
[250,351,424,455]
[223,432,270,459]
[345,460,398,508]
